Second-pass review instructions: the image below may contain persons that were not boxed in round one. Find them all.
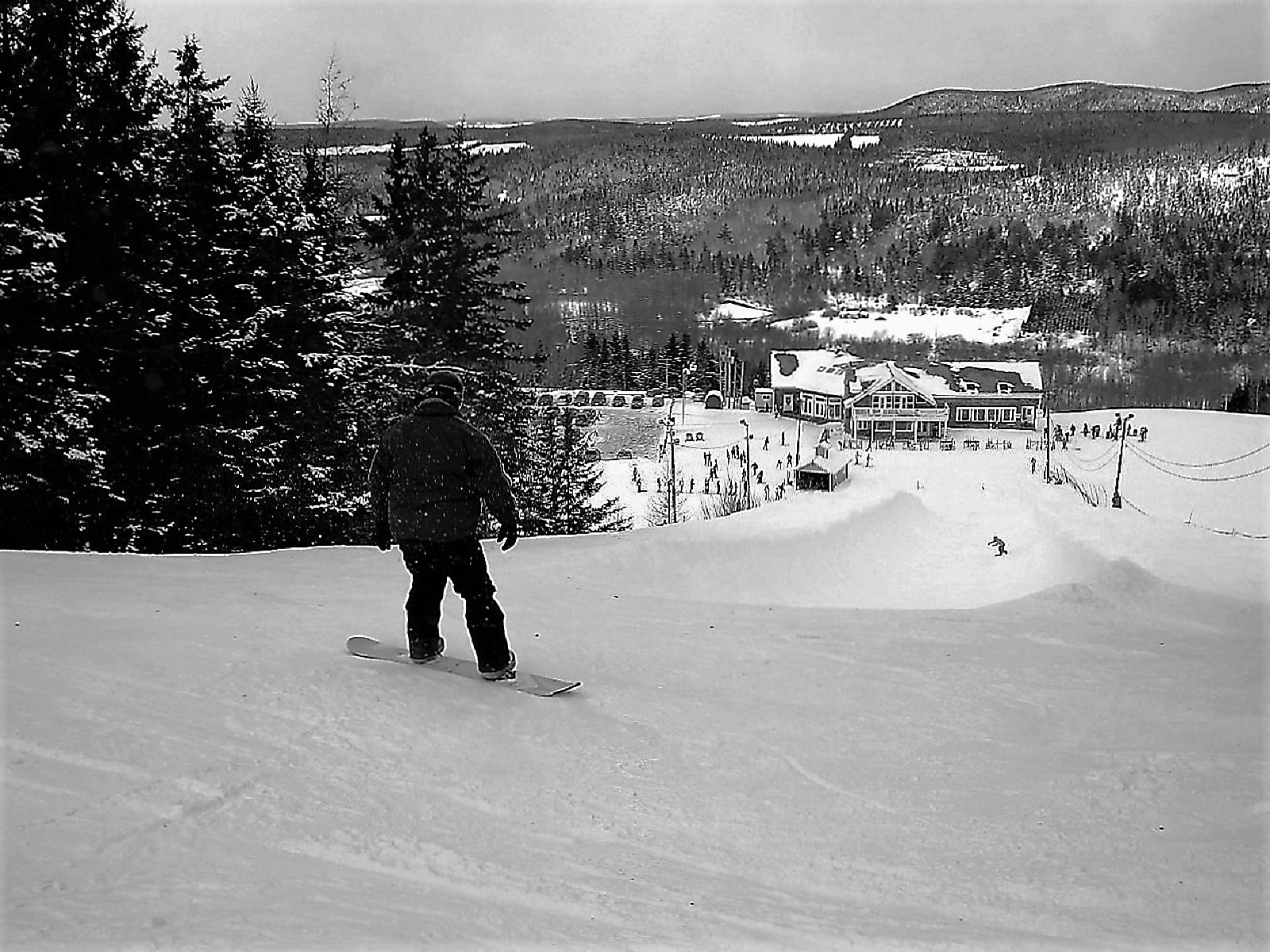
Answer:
[988,536,1008,556]
[752,436,792,500]
[368,371,519,682]
[631,467,667,493]
[1062,423,1148,451]
[1031,457,1036,474]
[679,444,745,494]
[838,441,873,467]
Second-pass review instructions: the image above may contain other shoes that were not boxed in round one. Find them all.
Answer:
[410,637,446,664]
[479,649,516,680]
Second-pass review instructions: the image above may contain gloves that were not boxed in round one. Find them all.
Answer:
[375,520,392,551]
[496,517,517,551]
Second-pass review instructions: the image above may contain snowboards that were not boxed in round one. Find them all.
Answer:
[346,636,583,697]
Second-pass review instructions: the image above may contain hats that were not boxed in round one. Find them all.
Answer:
[423,369,465,402]
